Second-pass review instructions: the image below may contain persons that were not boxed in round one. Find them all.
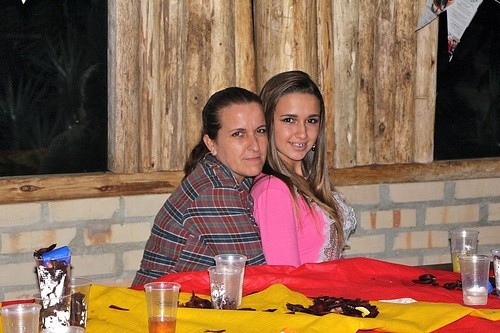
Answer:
[131,87,268,285]
[250,71,356,267]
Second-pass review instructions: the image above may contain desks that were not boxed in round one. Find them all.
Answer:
[0,262,500,333]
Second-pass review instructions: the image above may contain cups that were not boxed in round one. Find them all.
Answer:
[449,229,479,272]
[490,250,500,296]
[1,304,42,333]
[208,266,241,310]
[144,282,181,333]
[0,297,35,308]
[41,326,85,333]
[459,255,491,305]
[215,254,246,306]
[70,278,92,328]
[33,292,72,330]
